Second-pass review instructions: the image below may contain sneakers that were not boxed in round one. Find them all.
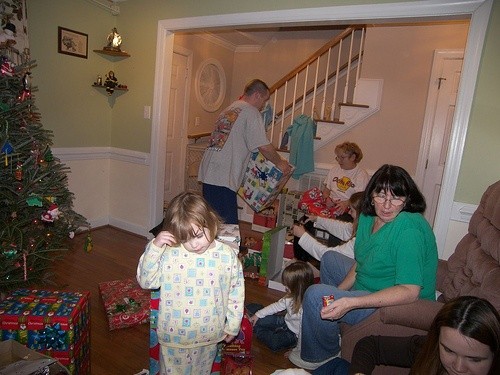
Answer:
[288,350,341,370]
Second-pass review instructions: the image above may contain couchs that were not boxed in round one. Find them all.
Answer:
[340,178,500,375]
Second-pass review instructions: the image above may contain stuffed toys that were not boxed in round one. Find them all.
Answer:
[40,203,60,222]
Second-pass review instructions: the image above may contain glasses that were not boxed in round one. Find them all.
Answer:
[335,156,349,161]
[371,194,408,206]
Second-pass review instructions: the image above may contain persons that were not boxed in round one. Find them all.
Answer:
[135,190,247,375]
[197,79,292,226]
[293,191,378,261]
[349,294,500,375]
[287,163,440,366]
[248,261,316,351]
[293,142,370,262]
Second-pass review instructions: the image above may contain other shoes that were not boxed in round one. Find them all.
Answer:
[246,303,264,312]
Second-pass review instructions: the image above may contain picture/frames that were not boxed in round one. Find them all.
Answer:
[58,27,89,59]
